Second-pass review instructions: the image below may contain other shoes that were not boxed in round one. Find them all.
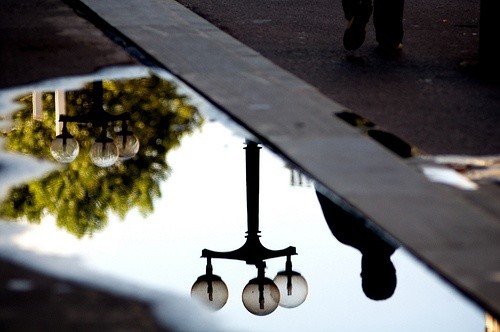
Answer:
[390,44,408,65]
[343,16,365,51]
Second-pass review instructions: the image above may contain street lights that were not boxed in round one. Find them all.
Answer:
[49,80,139,167]
[188,135,309,317]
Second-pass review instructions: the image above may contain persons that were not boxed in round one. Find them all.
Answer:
[316,188,402,300]
[342,0,404,65]
